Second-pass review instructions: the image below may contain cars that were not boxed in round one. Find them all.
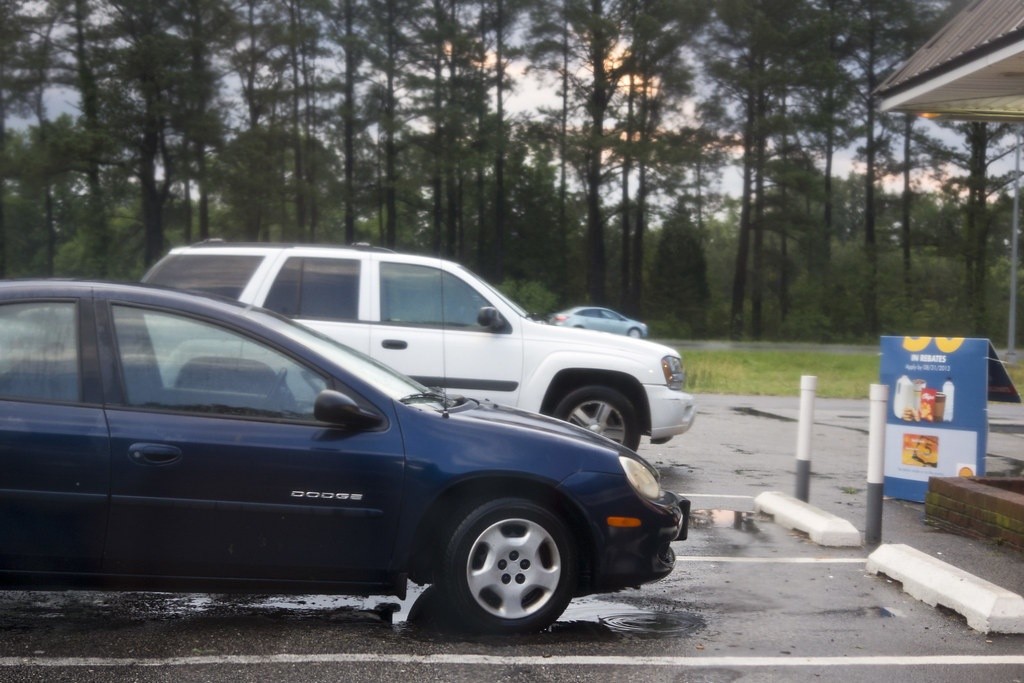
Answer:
[547,304,648,342]
[0,277,695,636]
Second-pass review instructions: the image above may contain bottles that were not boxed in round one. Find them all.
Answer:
[942,376,955,422]
[894,374,914,419]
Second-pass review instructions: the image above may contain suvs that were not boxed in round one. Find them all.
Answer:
[143,237,697,463]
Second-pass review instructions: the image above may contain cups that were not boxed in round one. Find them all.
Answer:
[934,392,946,423]
[912,380,926,412]
